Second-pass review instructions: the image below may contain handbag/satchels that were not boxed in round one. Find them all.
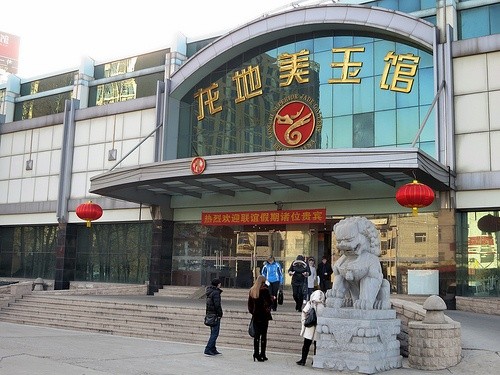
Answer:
[304,308,317,326]
[204,314,218,326]
[278,285,283,305]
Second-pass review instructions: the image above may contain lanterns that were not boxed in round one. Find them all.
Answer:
[76,201,102,227]
[396,180,435,216]
[478,214,500,232]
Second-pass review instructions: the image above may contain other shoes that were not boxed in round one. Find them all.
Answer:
[215,347,222,355]
[296,359,305,366]
[204,349,216,356]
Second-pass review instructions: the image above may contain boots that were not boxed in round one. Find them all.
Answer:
[253,338,264,361]
[260,339,268,360]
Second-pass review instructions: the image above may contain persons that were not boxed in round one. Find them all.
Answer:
[288,255,333,312]
[297,290,325,365]
[261,256,284,311]
[204,279,223,356]
[248,275,276,362]
